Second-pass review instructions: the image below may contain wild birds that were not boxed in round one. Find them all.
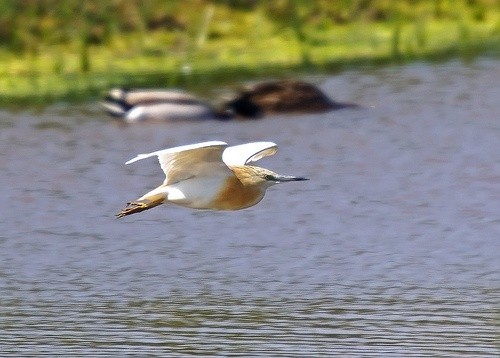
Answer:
[225,80,337,119]
[99,89,233,122]
[115,141,310,219]
